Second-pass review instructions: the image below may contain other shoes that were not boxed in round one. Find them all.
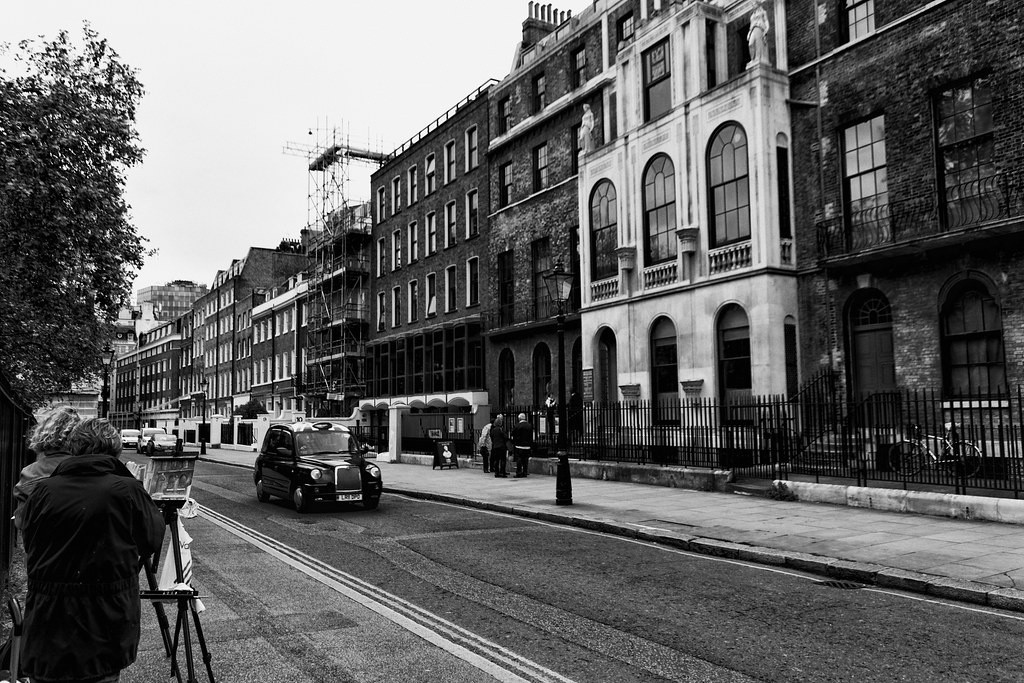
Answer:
[522,474,527,477]
[495,474,507,478]
[484,470,490,473]
[513,474,522,478]
[490,469,495,472]
[505,472,510,475]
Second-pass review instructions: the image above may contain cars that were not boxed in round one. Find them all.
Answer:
[146,434,178,457]
[119,428,140,449]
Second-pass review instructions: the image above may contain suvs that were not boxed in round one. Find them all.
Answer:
[137,427,166,454]
[254,421,382,514]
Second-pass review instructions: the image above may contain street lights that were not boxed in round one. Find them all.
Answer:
[540,264,574,505]
[99,340,115,418]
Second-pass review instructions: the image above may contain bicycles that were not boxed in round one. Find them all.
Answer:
[888,421,982,480]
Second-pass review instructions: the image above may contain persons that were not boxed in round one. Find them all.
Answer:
[511,413,532,478]
[13,406,80,530]
[478,414,510,478]
[22,417,165,683]
[746,1,769,64]
[579,104,594,154]
